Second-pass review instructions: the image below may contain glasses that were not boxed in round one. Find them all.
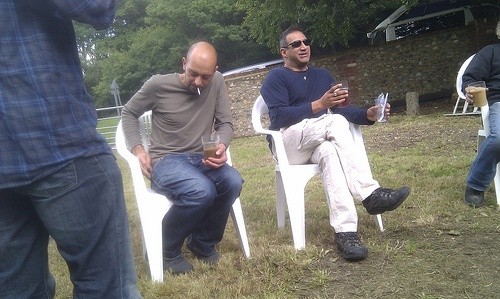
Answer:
[285,39,311,48]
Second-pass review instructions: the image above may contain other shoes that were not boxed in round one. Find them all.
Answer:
[465,186,484,208]
[163,255,194,274]
[195,250,219,262]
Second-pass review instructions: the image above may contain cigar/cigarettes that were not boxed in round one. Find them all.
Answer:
[197,88,201,95]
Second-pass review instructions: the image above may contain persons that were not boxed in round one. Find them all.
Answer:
[259,28,410,260]
[122,41,244,273]
[0,0,143,299]
[461,20,500,207]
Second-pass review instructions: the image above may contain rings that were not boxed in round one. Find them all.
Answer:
[334,92,337,96]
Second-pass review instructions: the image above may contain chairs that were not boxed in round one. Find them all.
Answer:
[115,110,252,284]
[251,95,383,252]
[455,53,500,205]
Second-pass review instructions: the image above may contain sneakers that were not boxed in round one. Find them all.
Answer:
[362,187,410,215]
[334,231,368,259]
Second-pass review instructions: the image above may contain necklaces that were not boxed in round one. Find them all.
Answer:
[284,65,311,81]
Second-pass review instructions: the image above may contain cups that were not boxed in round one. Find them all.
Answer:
[201,135,221,161]
[330,80,349,108]
[375,99,388,122]
[467,81,488,107]
